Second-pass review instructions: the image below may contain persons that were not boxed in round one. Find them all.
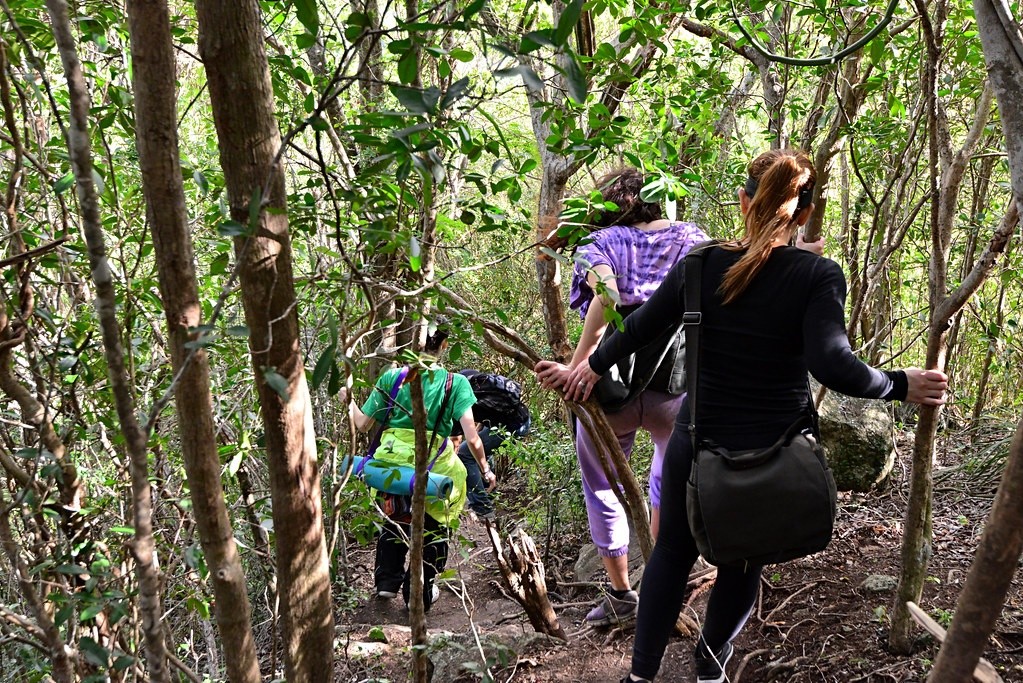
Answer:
[536,169,710,627]
[560,147,949,683]
[336,312,498,612]
[445,365,528,521]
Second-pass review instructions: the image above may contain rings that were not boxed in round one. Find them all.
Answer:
[578,381,585,387]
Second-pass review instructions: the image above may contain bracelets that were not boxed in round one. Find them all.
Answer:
[342,397,355,402]
[479,468,490,474]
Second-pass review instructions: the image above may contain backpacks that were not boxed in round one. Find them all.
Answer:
[461,373,524,416]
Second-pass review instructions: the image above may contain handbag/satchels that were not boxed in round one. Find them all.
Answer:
[686,428,837,567]
[374,492,413,525]
[593,303,689,415]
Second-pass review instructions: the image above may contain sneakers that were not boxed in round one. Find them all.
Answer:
[696,642,735,683]
[407,584,439,611]
[377,581,402,599]
[587,590,641,625]
